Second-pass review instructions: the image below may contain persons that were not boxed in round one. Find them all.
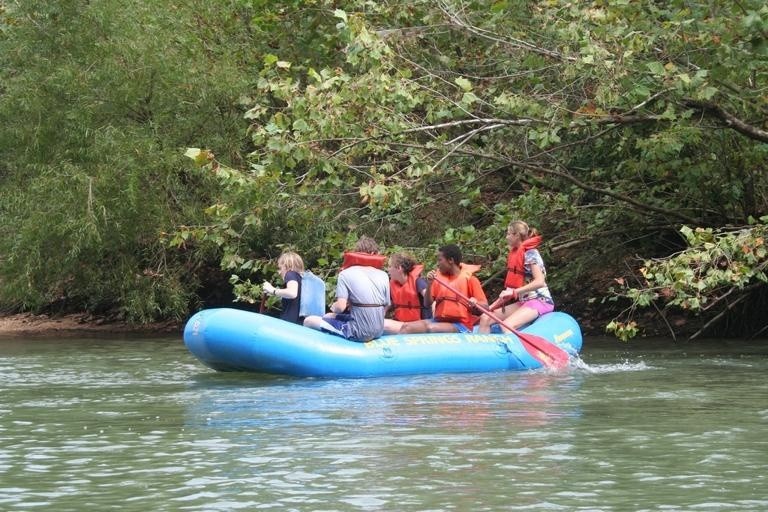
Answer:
[302,234,391,343]
[476,220,555,334]
[262,252,306,326]
[400,244,490,336]
[382,251,430,336]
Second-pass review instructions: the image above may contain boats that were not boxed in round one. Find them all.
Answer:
[183,308,582,377]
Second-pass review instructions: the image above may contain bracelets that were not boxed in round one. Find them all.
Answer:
[273,288,278,295]
[512,289,517,296]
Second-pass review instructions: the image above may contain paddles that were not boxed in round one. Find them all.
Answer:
[433,276,570,370]
